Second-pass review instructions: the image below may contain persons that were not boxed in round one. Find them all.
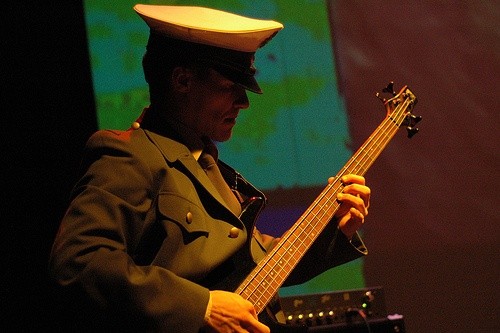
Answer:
[48,2,372,333]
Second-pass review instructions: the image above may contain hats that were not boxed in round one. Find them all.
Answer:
[134,4,284,94]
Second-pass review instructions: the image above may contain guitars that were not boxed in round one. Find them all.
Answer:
[232,81,422,316]
